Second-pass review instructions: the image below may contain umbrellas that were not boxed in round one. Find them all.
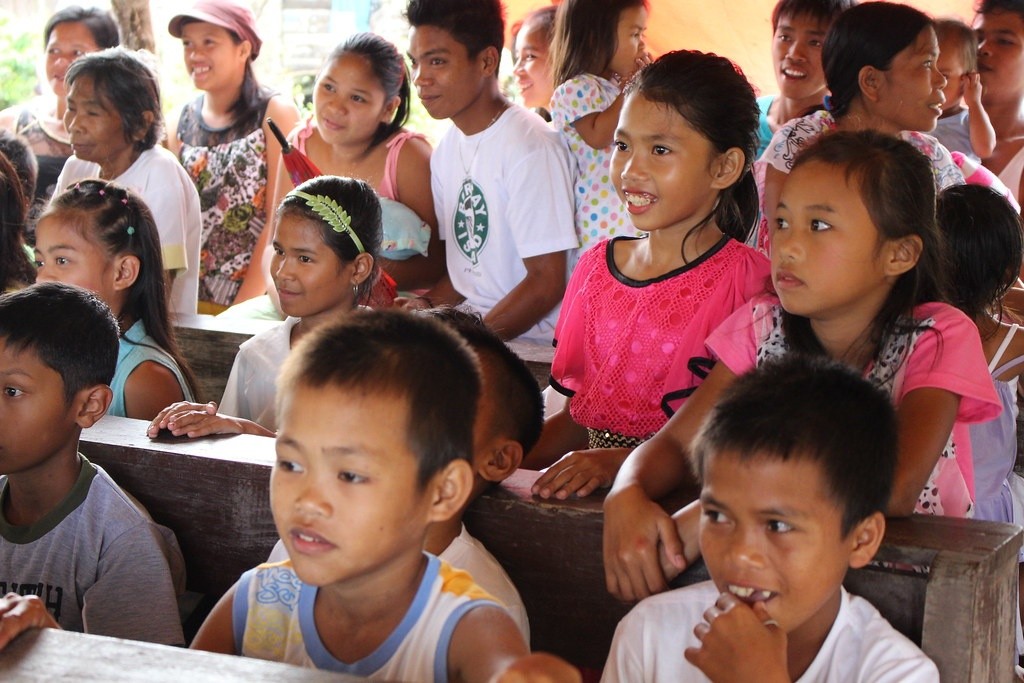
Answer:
[267,117,399,309]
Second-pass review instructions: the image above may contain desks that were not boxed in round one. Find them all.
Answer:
[0,312,1024,683]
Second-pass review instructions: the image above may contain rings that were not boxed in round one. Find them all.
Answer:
[761,619,778,629]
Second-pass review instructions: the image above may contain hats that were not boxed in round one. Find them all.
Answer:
[168,1,261,63]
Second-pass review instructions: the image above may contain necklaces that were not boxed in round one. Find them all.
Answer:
[459,99,509,183]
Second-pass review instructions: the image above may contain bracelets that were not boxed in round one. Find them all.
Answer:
[415,295,435,309]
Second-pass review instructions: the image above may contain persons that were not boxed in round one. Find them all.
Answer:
[0,0,1024,683]
